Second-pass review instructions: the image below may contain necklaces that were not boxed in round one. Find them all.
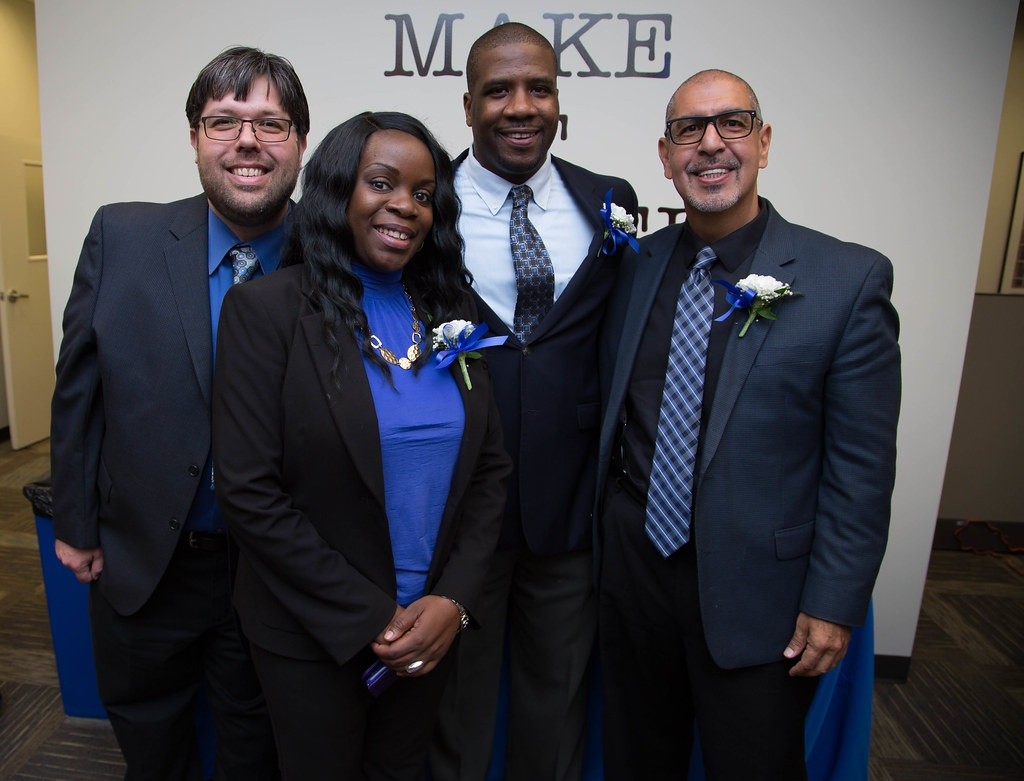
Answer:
[353,280,424,370]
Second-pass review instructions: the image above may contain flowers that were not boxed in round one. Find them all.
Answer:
[430,319,482,390]
[733,273,805,339]
[595,202,638,257]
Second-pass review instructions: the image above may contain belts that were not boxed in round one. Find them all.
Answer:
[177,530,239,554]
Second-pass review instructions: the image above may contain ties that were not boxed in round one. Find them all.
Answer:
[228,245,261,284]
[507,184,555,343]
[643,245,718,561]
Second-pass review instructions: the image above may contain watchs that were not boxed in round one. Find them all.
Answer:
[439,594,474,634]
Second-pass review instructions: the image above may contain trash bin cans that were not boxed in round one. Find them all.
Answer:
[29,478,121,719]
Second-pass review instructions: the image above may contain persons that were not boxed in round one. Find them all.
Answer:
[586,68,903,781]
[438,22,639,778]
[208,110,514,780]
[50,47,318,780]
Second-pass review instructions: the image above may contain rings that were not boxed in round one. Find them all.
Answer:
[406,658,425,674]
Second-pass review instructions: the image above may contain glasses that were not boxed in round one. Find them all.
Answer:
[663,109,765,145]
[194,115,300,143]
